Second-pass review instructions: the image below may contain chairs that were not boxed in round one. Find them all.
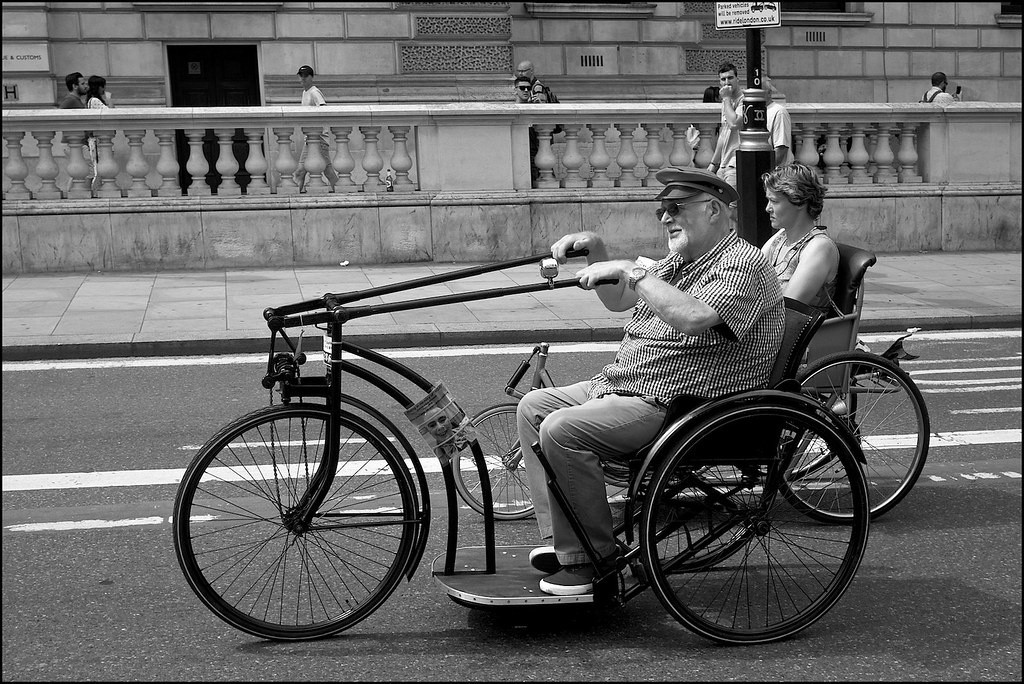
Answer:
[629,298,824,468]
[798,243,879,389]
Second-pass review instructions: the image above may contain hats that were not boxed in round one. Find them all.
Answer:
[654,167,740,208]
[297,66,314,75]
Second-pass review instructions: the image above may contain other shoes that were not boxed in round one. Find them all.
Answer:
[539,564,594,595]
[529,536,561,574]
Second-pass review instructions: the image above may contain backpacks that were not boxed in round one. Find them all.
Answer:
[533,82,560,104]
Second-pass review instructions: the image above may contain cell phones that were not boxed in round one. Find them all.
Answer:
[956,85,962,94]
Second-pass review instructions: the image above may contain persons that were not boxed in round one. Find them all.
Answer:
[58,72,95,191]
[516,166,785,595]
[693,63,794,231]
[513,60,557,189]
[294,64,338,192]
[922,72,962,103]
[84,75,115,189]
[761,159,840,316]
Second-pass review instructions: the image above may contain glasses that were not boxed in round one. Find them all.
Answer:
[515,85,532,91]
[516,68,531,74]
[298,73,311,78]
[944,82,949,86]
[655,200,711,221]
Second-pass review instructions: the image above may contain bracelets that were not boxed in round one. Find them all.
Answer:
[710,162,720,167]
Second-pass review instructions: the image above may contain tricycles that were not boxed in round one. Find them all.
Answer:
[171,245,932,643]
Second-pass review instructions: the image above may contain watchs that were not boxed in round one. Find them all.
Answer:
[628,267,649,292]
